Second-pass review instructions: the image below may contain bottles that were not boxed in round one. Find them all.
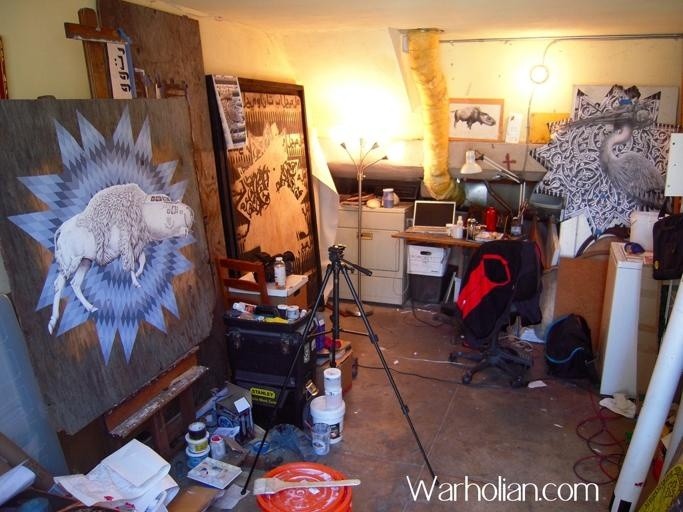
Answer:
[455,215,463,239]
[325,391,343,410]
[382,189,393,207]
[467,218,471,241]
[186,446,210,469]
[288,305,300,320]
[188,421,206,440]
[186,432,210,453]
[274,257,286,289]
[471,218,476,241]
[324,367,342,392]
[233,301,257,314]
[278,304,287,319]
[210,435,226,460]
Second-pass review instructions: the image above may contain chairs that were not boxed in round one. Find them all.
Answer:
[209,256,271,311]
[440,239,538,388]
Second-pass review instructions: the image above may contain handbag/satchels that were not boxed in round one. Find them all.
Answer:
[543,312,598,379]
[652,197,683,281]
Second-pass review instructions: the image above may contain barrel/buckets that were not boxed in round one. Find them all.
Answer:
[256,461,353,512]
[309,395,346,446]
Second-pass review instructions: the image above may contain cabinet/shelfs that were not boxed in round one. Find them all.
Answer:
[333,194,413,315]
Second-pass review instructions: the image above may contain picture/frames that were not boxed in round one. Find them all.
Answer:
[202,72,325,318]
[6,99,216,425]
[448,98,505,143]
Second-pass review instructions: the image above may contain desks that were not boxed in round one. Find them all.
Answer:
[390,223,529,326]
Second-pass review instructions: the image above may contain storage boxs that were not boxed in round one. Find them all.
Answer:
[312,343,354,397]
[404,243,452,277]
[226,272,309,312]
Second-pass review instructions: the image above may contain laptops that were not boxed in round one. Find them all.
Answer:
[407,199,457,235]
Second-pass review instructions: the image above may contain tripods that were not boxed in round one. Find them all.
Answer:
[238,242,438,495]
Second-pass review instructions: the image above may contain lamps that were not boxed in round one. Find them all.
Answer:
[460,149,526,239]
[330,120,402,316]
